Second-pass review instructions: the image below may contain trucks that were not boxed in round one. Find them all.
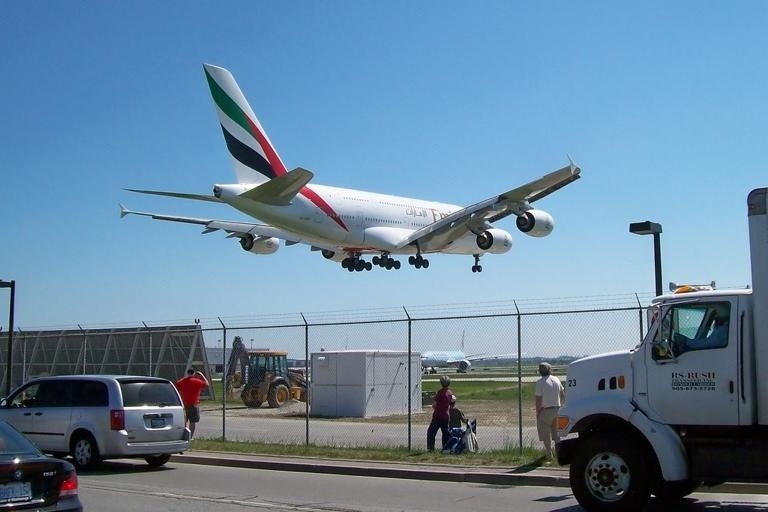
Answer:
[553,184,766,509]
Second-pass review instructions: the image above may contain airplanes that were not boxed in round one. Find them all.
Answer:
[118,62,590,273]
[422,328,528,375]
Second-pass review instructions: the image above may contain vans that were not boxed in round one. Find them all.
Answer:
[1,374,193,469]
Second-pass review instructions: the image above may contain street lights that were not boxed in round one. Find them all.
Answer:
[0,278,17,398]
[629,220,666,299]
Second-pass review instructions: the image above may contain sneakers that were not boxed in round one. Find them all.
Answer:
[544,453,553,461]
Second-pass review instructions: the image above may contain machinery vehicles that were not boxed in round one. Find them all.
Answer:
[222,334,310,409]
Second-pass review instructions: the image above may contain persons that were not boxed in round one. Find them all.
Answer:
[535,361,566,461]
[450,394,468,427]
[673,302,731,350]
[175,367,209,439]
[426,375,454,453]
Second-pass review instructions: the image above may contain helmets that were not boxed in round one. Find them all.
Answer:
[440,376,450,387]
[449,395,457,404]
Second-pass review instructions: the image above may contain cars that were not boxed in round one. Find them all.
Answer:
[1,419,83,512]
